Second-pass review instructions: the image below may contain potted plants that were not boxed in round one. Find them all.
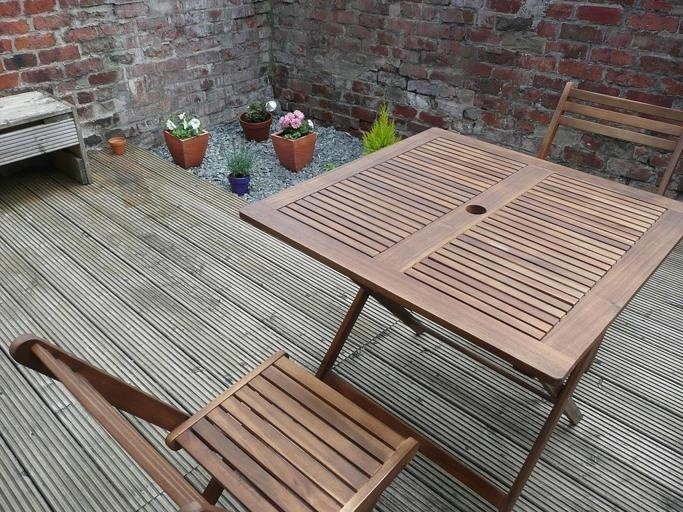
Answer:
[226,144,254,197]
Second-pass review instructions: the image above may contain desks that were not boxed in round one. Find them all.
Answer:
[236,121,682,512]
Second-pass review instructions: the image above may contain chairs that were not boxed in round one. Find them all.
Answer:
[8,330,424,512]
[539,76,683,197]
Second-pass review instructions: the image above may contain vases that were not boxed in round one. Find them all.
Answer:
[106,132,132,157]
[238,110,273,144]
[272,130,321,171]
[163,130,209,172]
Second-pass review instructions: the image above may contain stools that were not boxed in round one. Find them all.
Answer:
[0,90,92,187]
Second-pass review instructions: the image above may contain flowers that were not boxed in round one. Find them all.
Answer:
[244,99,278,121]
[162,109,206,141]
[277,109,315,140]
